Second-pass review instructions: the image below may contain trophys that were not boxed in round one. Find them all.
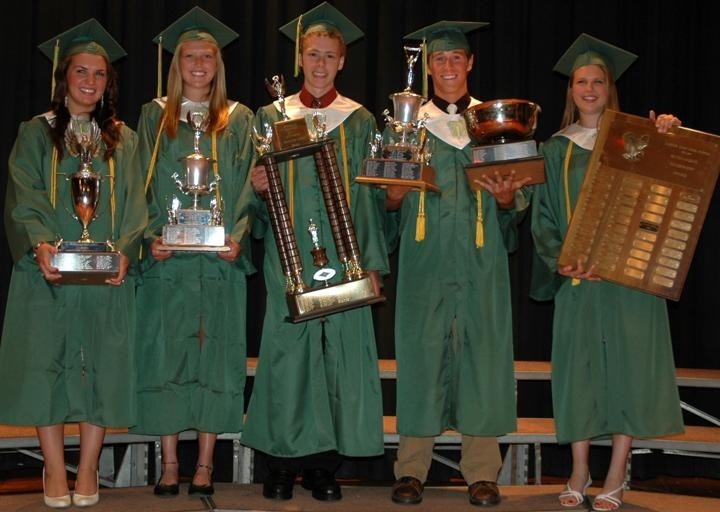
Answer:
[46,116,120,285]
[248,73,387,325]
[460,96,547,194]
[159,110,231,254]
[354,47,442,193]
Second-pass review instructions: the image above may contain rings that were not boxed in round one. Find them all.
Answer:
[122,278,126,284]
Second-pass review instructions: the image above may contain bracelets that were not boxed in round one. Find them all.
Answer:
[31,241,46,262]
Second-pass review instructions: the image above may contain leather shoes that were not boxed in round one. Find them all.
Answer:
[468,481,501,507]
[262,471,295,500]
[73,466,100,509]
[188,464,214,496]
[390,476,423,504]
[42,466,71,507]
[301,468,342,502]
[154,462,181,498]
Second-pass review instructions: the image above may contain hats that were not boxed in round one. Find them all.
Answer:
[153,6,239,99]
[38,19,129,103]
[278,2,365,79]
[402,21,490,101]
[552,33,637,85]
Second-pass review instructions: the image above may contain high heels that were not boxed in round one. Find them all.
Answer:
[592,483,625,511]
[558,471,593,507]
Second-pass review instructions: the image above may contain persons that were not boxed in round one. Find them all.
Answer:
[1,17,138,510]
[238,0,385,500]
[531,30,685,511]
[375,19,534,505]
[126,5,258,501]
